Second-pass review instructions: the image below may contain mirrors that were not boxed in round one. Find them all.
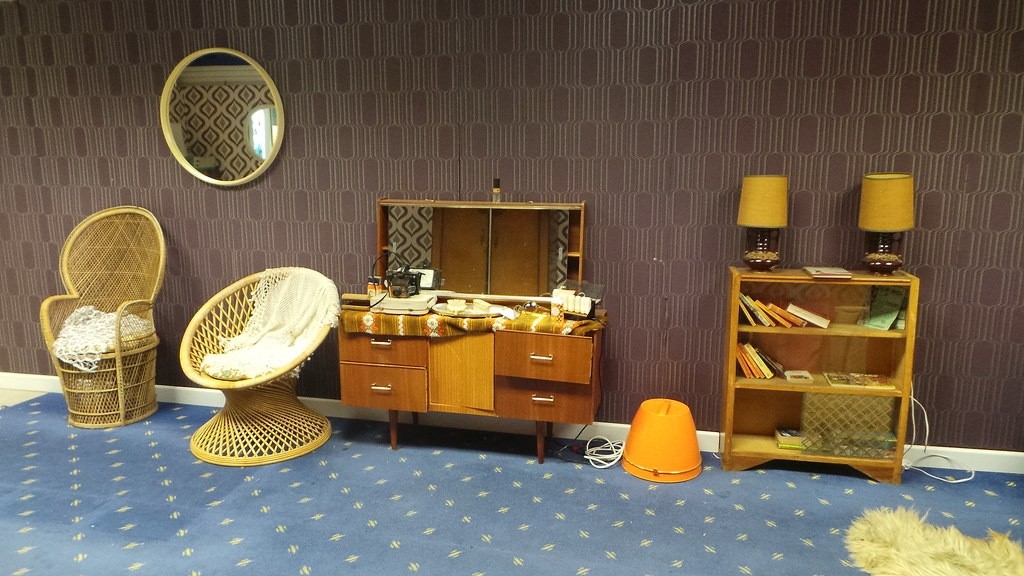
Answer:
[161,48,285,185]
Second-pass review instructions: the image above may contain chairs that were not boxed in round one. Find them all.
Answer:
[179,266,338,467]
[40,204,167,430]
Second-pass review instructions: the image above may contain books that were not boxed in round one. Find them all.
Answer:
[858,286,909,331]
[738,292,831,329]
[822,370,897,390]
[776,426,897,458]
[736,341,813,383]
[803,266,853,280]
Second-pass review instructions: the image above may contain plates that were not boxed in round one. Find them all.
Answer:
[432,304,505,317]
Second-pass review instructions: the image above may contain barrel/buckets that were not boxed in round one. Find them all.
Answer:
[620,398,703,483]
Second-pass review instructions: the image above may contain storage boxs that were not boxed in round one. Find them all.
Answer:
[193,156,215,168]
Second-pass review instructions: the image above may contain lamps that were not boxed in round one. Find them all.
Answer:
[857,171,916,278]
[737,174,789,271]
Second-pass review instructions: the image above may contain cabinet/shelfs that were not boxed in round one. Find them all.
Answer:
[718,266,920,485]
[340,198,603,463]
